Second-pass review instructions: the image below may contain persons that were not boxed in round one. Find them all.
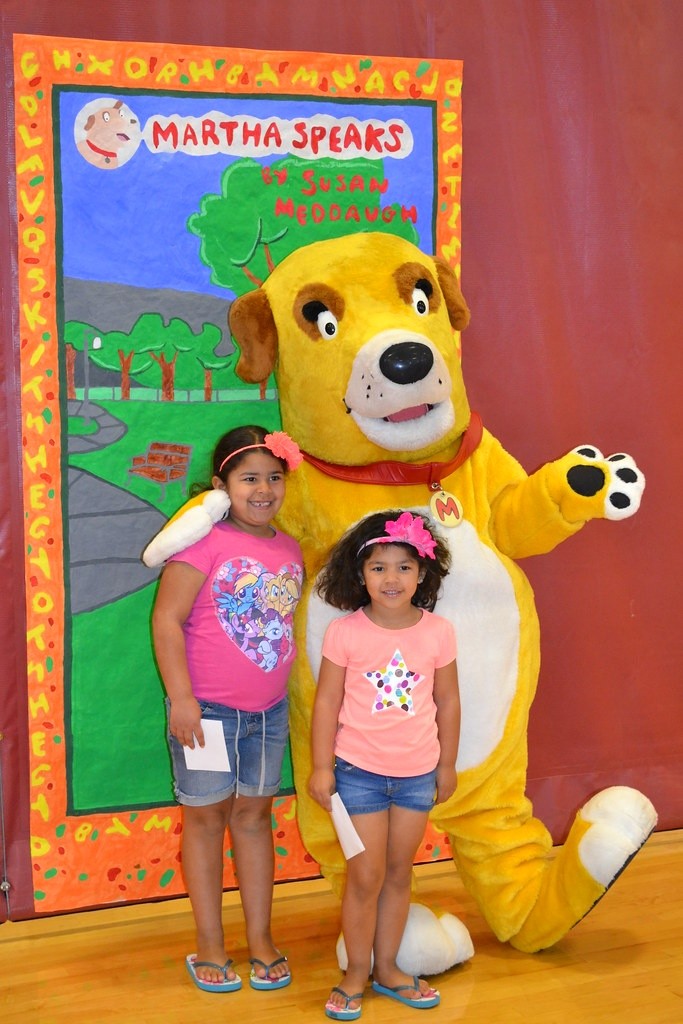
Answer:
[307,509,459,1021]
[150,425,306,992]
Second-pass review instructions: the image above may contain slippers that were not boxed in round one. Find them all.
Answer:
[371,974,440,1009]
[325,984,365,1021]
[186,952,243,992]
[249,948,292,990]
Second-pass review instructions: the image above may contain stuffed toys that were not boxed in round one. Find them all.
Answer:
[143,232,658,978]
